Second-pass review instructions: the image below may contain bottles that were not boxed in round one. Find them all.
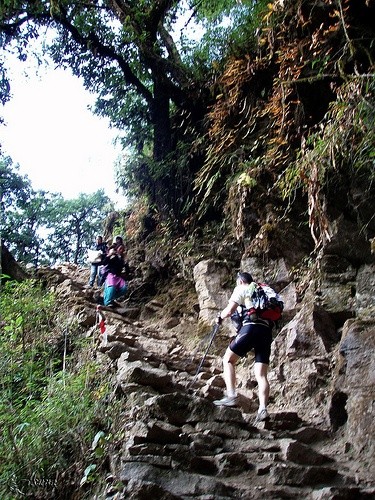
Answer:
[245,297,256,320]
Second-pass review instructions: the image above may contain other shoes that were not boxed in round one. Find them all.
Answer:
[106,304,115,308]
[85,285,93,288]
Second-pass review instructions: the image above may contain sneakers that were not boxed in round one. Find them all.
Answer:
[255,409,268,422]
[213,395,239,406]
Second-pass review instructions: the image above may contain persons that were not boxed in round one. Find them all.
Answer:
[88,236,126,308]
[212,272,275,423]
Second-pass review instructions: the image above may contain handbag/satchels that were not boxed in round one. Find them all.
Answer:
[88,249,103,263]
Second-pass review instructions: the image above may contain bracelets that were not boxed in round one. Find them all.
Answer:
[215,316,223,325]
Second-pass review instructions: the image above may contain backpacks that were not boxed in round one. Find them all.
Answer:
[250,282,284,321]
